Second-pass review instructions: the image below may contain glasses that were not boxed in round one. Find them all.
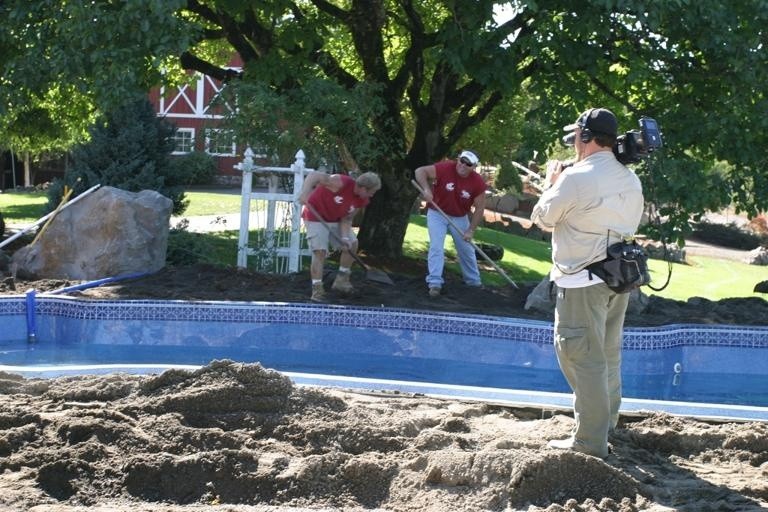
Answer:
[459,157,473,167]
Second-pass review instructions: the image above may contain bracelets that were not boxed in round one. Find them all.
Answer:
[340,237,351,240]
[468,229,475,234]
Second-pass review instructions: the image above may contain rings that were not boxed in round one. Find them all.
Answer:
[466,239,469,242]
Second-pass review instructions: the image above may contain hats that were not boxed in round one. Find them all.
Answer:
[561,107,618,137]
[457,150,479,165]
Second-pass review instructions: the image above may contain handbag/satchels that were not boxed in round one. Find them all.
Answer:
[581,237,653,295]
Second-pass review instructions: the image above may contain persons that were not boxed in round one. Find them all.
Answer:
[416,150,488,299]
[296,169,382,303]
[532,107,647,457]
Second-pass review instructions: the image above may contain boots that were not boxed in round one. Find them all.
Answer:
[329,270,361,299]
[309,281,329,304]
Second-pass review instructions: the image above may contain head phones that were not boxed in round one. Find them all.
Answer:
[575,106,598,144]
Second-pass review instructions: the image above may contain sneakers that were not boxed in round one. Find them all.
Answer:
[427,286,442,298]
[543,436,576,453]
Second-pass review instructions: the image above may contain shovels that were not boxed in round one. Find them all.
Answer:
[13,187,73,282]
[306,204,395,285]
